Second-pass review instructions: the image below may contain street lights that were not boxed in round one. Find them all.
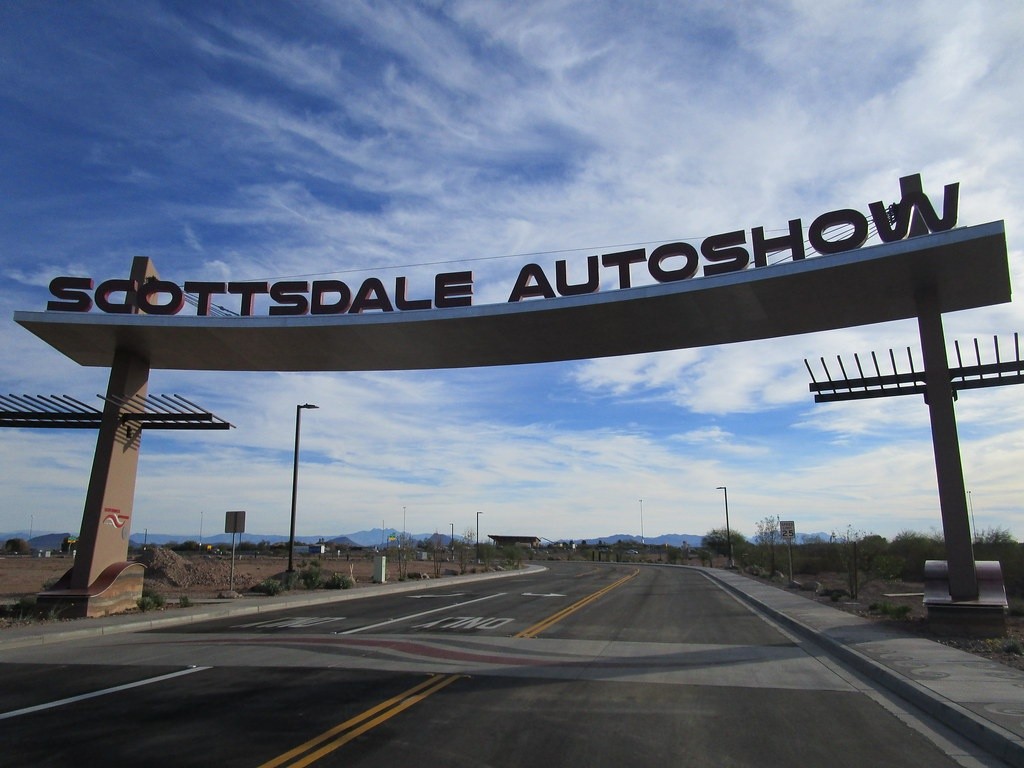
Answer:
[639,499,643,543]
[477,512,483,547]
[450,523,453,546]
[286,402,319,572]
[717,487,732,559]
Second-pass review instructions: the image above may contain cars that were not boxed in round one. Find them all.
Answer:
[626,550,638,554]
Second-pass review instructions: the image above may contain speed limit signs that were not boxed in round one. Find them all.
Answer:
[780,521,795,539]
